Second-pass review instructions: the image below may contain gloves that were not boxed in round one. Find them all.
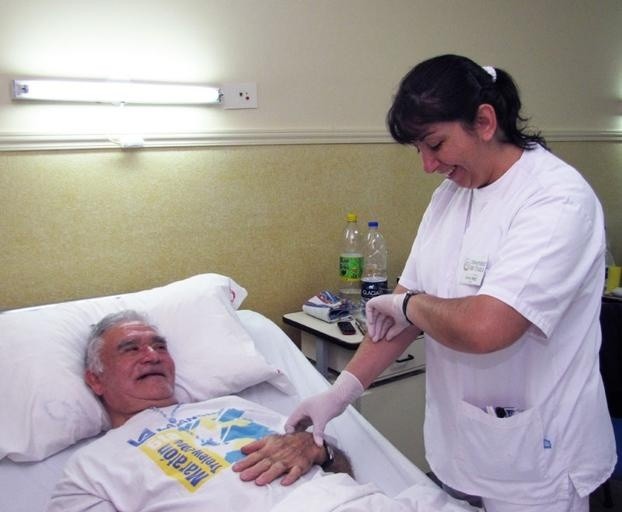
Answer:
[364,292,411,342]
[283,370,365,449]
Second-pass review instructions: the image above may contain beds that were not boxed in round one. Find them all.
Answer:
[0,313,481,512]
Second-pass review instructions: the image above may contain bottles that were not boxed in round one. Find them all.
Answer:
[338,212,364,313]
[360,221,388,318]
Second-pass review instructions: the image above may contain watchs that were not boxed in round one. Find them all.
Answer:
[321,439,334,471]
[402,288,426,324]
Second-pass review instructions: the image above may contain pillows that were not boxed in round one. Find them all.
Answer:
[0,273,300,470]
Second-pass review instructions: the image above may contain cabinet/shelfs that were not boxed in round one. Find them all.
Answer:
[283,303,435,493]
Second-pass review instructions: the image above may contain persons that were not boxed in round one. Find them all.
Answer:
[44,307,415,512]
[283,53,617,512]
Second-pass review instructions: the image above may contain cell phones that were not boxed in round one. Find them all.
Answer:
[337,321,356,335]
